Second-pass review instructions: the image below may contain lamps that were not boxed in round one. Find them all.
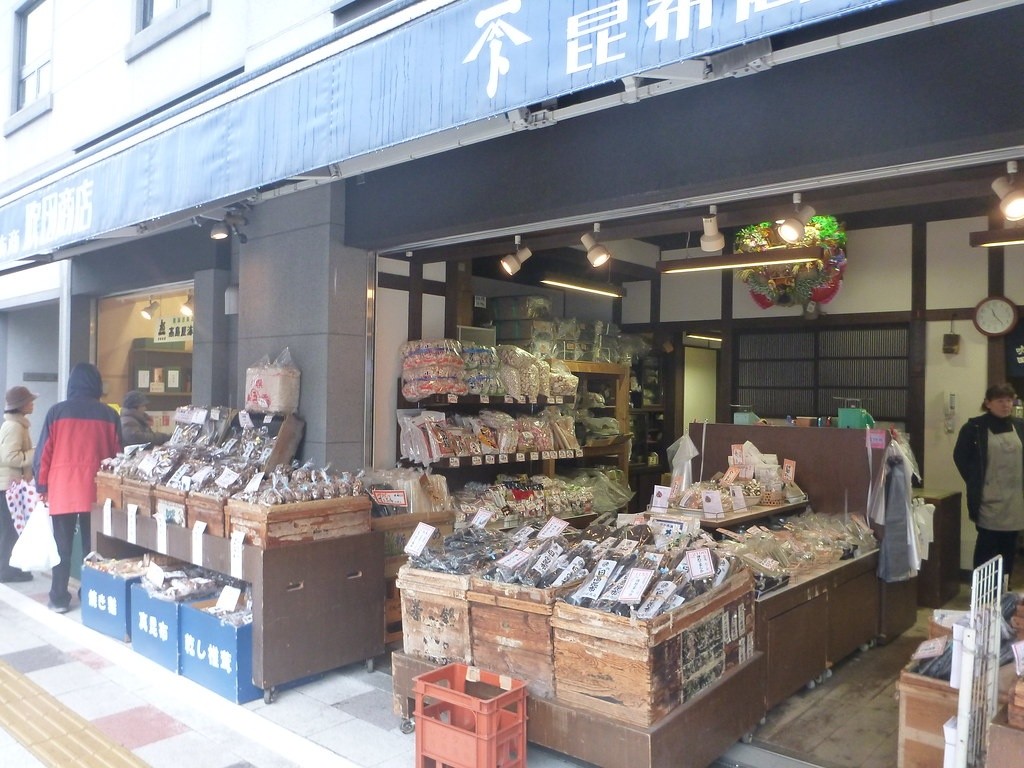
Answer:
[180,288,195,316]
[686,330,723,341]
[500,234,532,275]
[539,268,627,297]
[210,221,229,240]
[991,159,1024,222]
[968,225,1024,248]
[141,295,160,320]
[657,245,827,275]
[777,192,815,244]
[699,205,726,252]
[579,222,611,267]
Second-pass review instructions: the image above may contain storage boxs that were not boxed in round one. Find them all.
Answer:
[395,562,497,667]
[94,404,374,552]
[79,554,195,643]
[546,565,755,728]
[179,586,326,706]
[410,662,531,768]
[465,564,588,702]
[130,560,252,676]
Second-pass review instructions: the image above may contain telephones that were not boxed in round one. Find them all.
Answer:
[943,392,957,418]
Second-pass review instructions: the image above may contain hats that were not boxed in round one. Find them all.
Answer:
[124,391,150,408]
[4,386,40,411]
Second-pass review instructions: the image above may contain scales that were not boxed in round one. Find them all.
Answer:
[833,395,876,430]
[729,403,761,426]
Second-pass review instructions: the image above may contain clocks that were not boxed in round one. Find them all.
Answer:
[971,295,1020,336]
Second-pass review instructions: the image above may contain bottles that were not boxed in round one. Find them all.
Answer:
[648,450,658,468]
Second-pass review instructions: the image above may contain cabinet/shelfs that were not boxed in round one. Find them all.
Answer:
[389,650,771,768]
[687,421,920,646]
[649,495,882,727]
[893,596,1023,767]
[91,503,387,704]
[539,357,632,515]
[629,348,673,514]
[369,509,457,644]
[911,487,962,610]
[397,376,600,530]
[133,337,193,435]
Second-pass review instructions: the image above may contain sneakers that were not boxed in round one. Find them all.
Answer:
[49,591,72,613]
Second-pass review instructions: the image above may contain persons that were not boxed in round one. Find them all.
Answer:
[31,364,124,615]
[0,386,38,583]
[120,390,173,455]
[953,383,1024,597]
